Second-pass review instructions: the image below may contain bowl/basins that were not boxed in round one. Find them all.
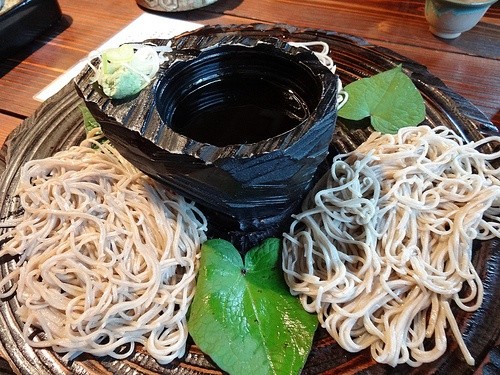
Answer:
[75,27,339,234]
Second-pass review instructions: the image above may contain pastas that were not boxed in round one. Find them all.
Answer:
[280,123,500,370]
[0,126,209,364]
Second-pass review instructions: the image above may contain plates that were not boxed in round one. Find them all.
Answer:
[1,21,500,375]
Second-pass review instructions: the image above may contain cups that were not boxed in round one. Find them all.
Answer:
[422,1,496,40]
